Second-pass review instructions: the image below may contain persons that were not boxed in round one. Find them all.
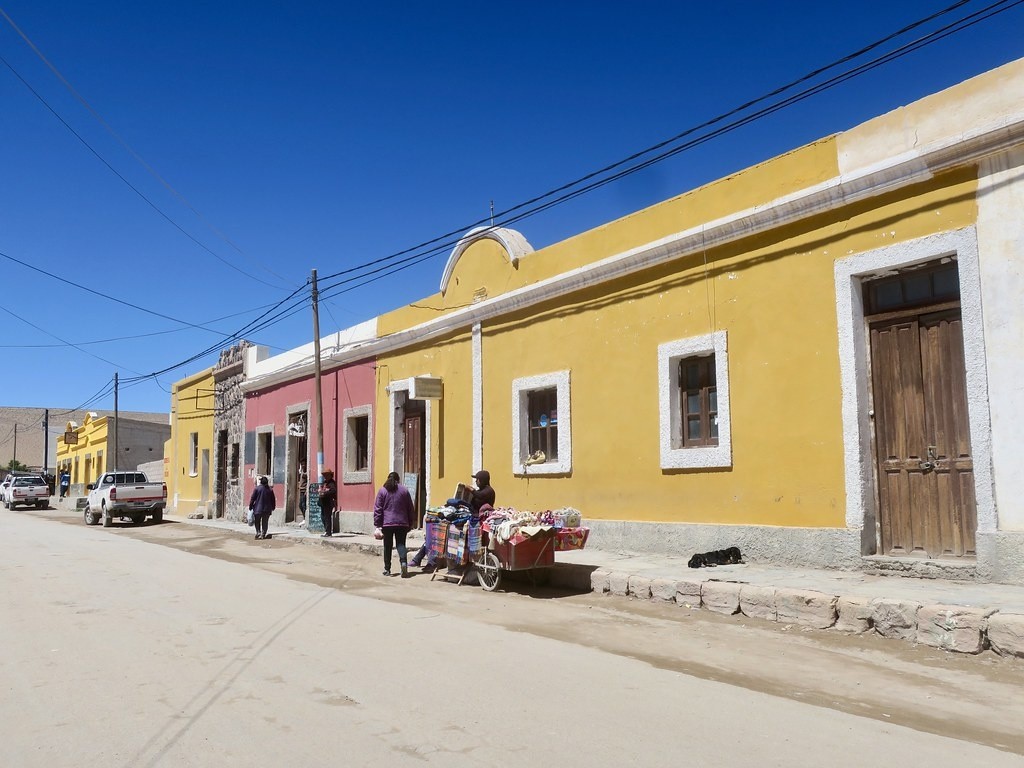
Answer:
[299,458,308,525]
[60,471,70,498]
[374,472,415,576]
[44,475,55,495]
[408,471,496,565]
[59,470,64,478]
[249,477,275,539]
[318,469,337,536]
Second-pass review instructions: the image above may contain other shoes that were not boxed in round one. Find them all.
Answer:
[261,533,265,540]
[401,563,408,578]
[382,570,391,576]
[255,531,261,538]
[320,532,332,538]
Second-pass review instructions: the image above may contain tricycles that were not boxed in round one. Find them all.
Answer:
[427,509,555,592]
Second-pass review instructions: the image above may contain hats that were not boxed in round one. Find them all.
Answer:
[471,470,490,484]
[259,477,268,480]
[320,467,334,475]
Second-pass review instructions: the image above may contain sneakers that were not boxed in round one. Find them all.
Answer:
[421,564,437,572]
[407,560,420,567]
[299,520,305,525]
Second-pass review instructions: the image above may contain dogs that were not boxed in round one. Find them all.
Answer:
[688,546,745,568]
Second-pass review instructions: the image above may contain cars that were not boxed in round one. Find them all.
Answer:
[0,472,34,500]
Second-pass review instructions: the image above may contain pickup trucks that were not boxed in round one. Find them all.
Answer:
[83,470,168,527]
[3,475,52,510]
